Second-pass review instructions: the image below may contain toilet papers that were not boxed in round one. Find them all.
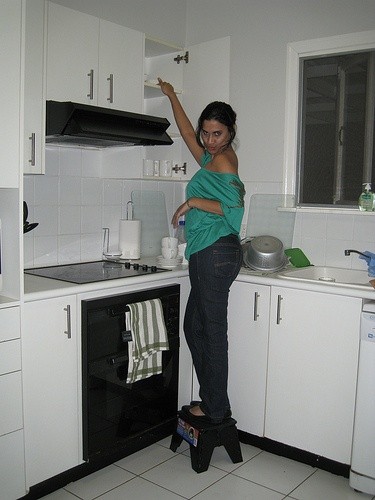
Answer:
[118,219,141,260]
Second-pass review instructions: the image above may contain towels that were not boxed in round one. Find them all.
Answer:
[125,298,170,384]
[359,250,375,278]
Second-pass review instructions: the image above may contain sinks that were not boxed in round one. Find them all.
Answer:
[277,266,375,291]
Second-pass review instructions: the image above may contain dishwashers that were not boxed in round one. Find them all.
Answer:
[349,300,375,495]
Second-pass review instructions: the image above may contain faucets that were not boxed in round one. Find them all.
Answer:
[345,249,371,261]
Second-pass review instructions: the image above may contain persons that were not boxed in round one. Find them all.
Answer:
[157,77,246,423]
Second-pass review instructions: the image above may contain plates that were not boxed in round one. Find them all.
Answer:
[157,257,183,267]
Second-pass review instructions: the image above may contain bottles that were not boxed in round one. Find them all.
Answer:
[175,221,187,245]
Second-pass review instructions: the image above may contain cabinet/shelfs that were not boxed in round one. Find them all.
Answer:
[191,276,363,480]
[22,0,48,176]
[0,1,26,500]
[46,0,145,115]
[142,33,231,137]
[25,294,82,492]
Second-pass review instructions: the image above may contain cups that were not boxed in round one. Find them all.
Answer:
[162,248,178,259]
[159,160,172,177]
[161,238,178,248]
[154,161,159,177]
[178,243,189,264]
[143,160,154,177]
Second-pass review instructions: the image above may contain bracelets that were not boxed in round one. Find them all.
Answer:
[186,199,193,210]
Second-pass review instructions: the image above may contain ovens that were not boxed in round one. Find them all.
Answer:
[81,283,180,461]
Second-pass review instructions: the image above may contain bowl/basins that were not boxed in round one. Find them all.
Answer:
[243,235,288,272]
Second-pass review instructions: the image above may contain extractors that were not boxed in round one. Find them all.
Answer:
[45,100,174,146]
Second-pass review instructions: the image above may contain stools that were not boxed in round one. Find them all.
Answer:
[169,413,243,473]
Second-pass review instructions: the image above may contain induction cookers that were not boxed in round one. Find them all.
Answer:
[24,260,171,284]
